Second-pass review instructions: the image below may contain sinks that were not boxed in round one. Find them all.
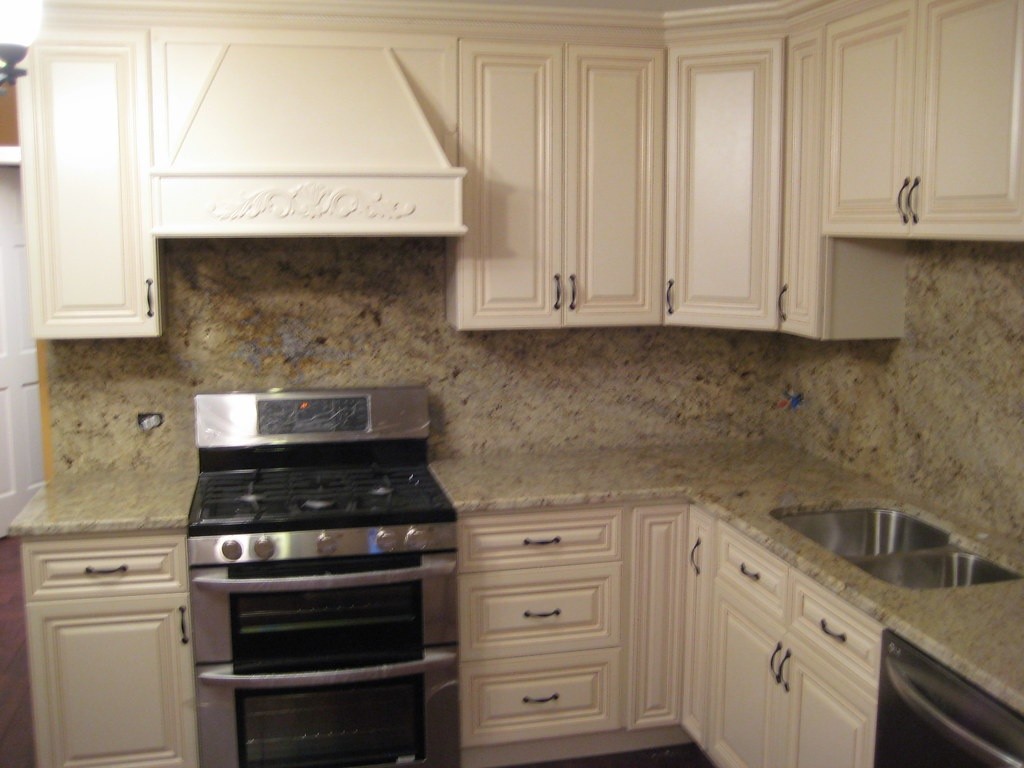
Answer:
[769,496,950,558]
[847,545,1024,591]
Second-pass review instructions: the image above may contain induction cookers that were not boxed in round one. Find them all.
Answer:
[187,387,458,565]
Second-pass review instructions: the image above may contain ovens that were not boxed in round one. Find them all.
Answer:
[190,553,459,768]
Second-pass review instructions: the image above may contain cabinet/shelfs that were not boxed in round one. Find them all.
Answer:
[19,535,202,768]
[669,23,905,342]
[820,0,1024,244]
[681,502,709,759]
[460,507,623,748]
[629,503,680,734]
[446,37,669,334]
[709,517,887,767]
[13,22,164,341]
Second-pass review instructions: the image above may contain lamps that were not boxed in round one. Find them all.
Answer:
[0,0,41,88]
